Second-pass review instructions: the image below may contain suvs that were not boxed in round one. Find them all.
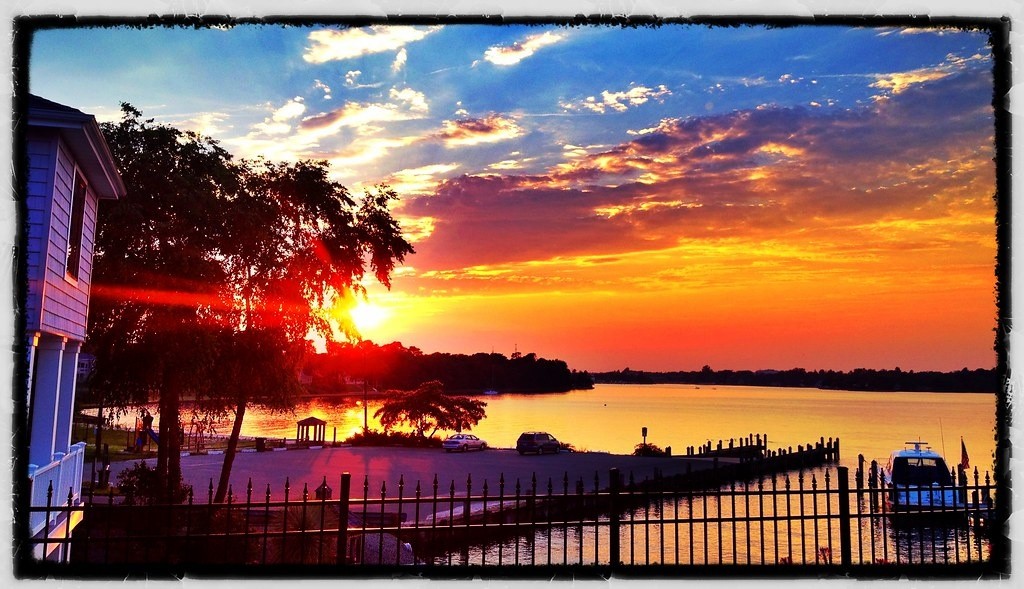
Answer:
[516,431,561,455]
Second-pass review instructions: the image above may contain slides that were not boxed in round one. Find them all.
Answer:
[148,429,159,445]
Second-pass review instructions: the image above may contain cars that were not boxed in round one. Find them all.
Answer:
[442,434,488,453]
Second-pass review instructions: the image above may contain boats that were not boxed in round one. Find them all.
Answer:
[866,437,968,524]
[483,390,499,396]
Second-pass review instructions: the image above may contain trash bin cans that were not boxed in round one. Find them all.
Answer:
[255,438,267,452]
